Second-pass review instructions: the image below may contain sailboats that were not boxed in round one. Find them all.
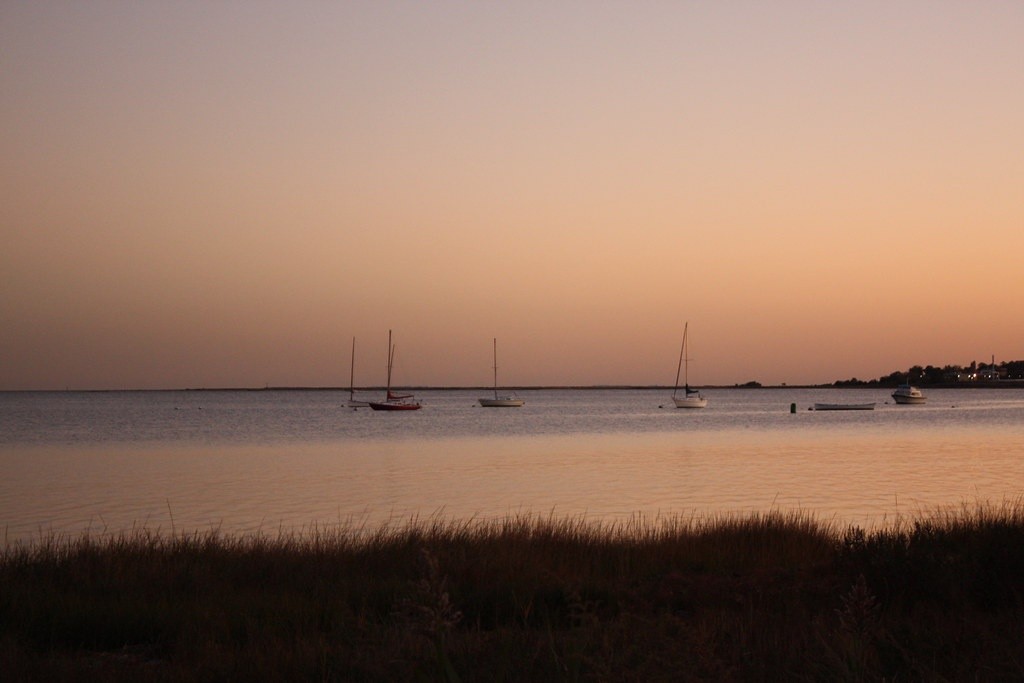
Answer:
[477,338,526,408]
[670,321,709,408]
[346,336,372,408]
[369,328,424,410]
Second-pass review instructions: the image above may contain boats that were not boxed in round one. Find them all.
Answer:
[890,377,928,404]
[813,402,877,410]
